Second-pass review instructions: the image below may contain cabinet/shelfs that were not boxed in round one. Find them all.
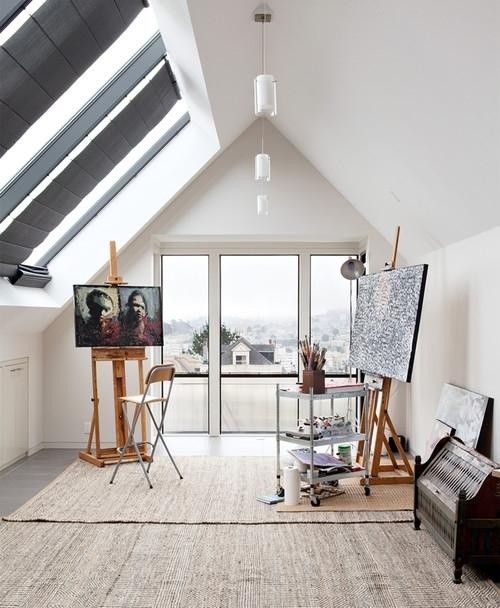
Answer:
[275,380,372,506]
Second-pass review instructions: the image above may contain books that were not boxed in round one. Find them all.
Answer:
[257,495,284,505]
[299,383,364,393]
[288,450,348,467]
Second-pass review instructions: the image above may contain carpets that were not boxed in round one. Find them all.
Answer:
[0,446,499,607]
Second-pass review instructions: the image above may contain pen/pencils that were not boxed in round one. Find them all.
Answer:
[298,336,327,370]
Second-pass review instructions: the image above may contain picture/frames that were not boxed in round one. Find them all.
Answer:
[72,283,163,348]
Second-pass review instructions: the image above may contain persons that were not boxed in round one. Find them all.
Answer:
[85,288,121,346]
[124,289,160,345]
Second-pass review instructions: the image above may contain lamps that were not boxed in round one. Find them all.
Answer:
[253,12,278,218]
[339,258,366,415]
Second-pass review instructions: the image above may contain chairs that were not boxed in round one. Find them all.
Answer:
[107,363,184,489]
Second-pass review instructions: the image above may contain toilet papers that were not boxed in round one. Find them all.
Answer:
[282,467,301,506]
